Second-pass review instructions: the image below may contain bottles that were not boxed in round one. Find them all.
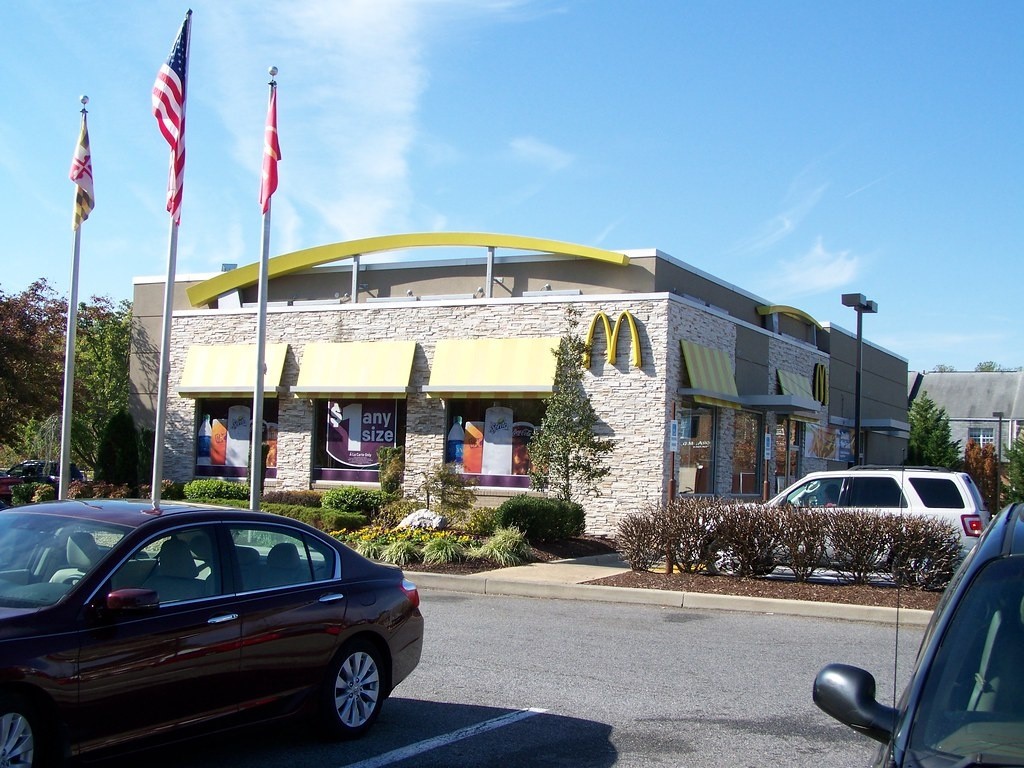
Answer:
[447,415,465,474]
[197,414,212,465]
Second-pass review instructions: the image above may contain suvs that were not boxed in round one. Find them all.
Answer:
[0,460,84,511]
[698,464,991,590]
[812,502,1024,768]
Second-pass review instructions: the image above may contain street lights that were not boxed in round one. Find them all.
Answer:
[841,293,878,465]
[992,412,1004,513]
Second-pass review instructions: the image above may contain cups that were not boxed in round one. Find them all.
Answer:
[226,405,250,467]
[249,419,268,467]
[463,421,484,473]
[265,423,278,467]
[482,403,513,475]
[210,419,228,465]
[513,422,534,475]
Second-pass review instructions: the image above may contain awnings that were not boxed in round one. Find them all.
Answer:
[288,338,418,402]
[177,341,288,400]
[422,335,562,397]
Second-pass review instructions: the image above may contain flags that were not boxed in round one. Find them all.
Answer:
[152,18,190,224]
[68,113,95,231]
[258,83,282,214]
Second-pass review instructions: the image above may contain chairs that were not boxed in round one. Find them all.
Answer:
[144,538,306,602]
[48,531,100,585]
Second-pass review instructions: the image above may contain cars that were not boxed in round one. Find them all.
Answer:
[0,499,424,768]
